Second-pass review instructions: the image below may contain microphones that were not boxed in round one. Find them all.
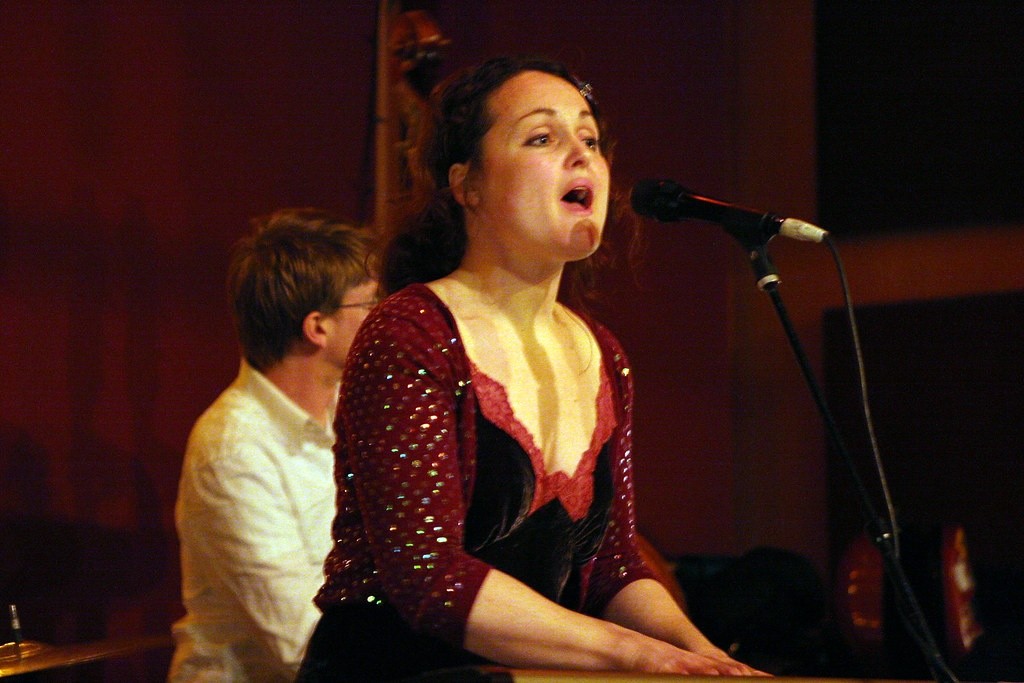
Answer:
[628,172,828,245]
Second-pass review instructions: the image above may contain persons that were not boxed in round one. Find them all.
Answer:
[295,53,774,683]
[169,208,381,683]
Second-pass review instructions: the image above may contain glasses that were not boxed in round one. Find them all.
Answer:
[329,297,381,312]
